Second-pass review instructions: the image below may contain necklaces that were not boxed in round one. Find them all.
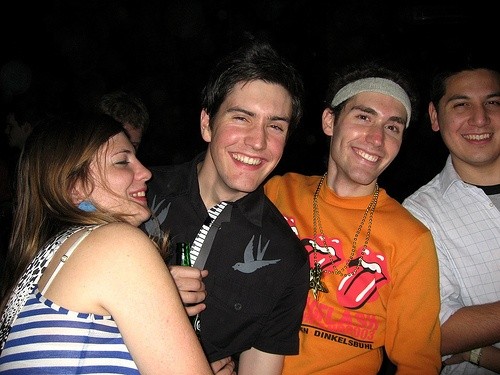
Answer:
[312,171,380,276]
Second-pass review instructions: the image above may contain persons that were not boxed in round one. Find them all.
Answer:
[4,103,47,149]
[401,51,500,375]
[0,91,216,375]
[264,56,441,375]
[137,42,311,375]
[96,91,149,152]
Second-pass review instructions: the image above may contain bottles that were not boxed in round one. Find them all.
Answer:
[174,242,201,338]
